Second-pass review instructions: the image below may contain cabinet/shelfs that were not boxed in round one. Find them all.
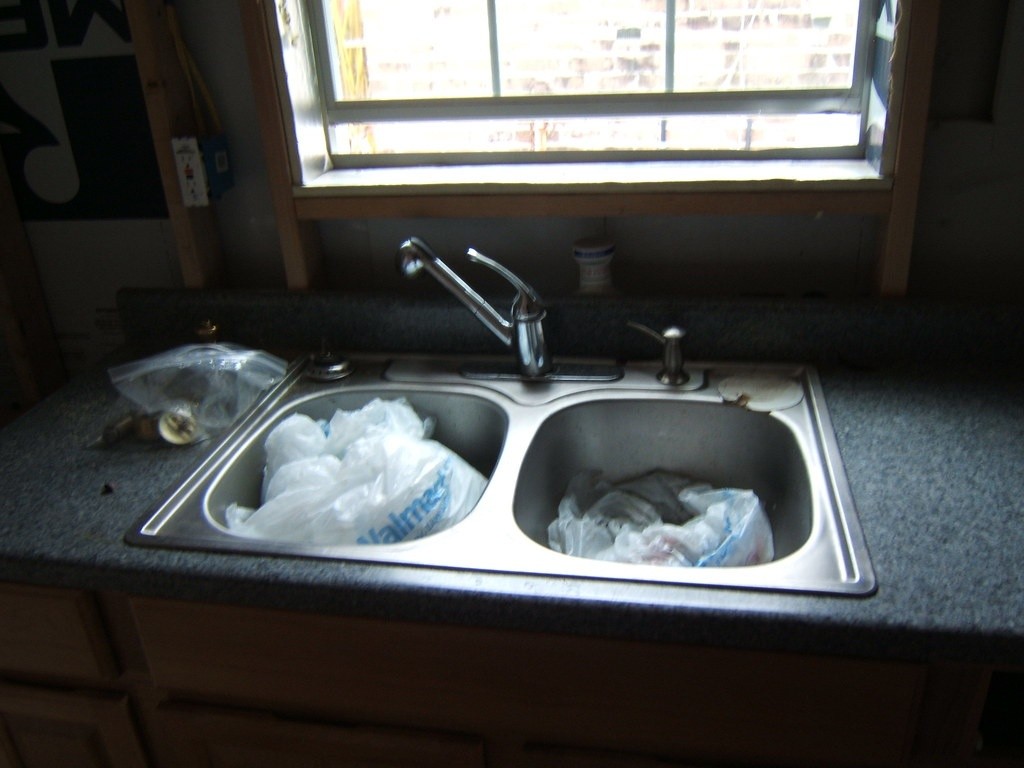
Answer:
[0,583,987,768]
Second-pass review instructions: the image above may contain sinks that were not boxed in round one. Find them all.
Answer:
[121,383,520,549]
[506,390,876,596]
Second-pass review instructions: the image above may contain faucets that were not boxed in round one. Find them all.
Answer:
[399,236,552,375]
